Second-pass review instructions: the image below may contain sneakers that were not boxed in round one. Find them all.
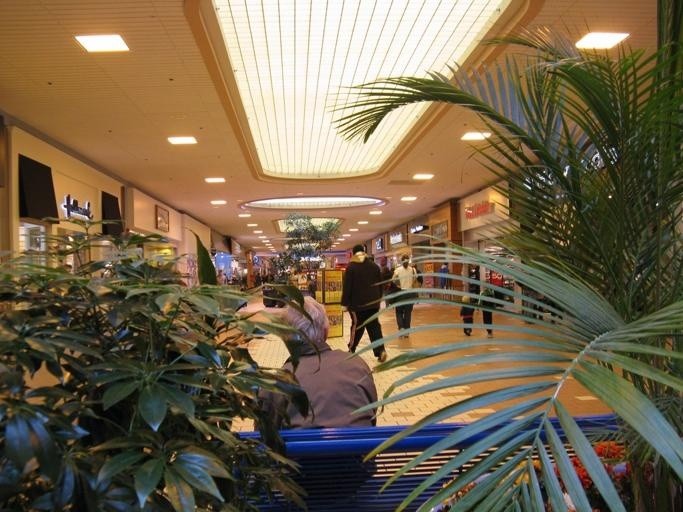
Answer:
[488,334,493,338]
[377,351,387,362]
[465,330,471,336]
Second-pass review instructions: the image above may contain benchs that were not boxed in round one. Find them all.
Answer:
[228,412,624,512]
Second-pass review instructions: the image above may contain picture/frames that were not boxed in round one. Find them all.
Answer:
[154,204,170,232]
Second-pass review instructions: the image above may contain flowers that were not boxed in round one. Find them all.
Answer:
[430,442,657,512]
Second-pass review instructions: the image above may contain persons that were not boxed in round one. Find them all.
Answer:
[217,267,240,284]
